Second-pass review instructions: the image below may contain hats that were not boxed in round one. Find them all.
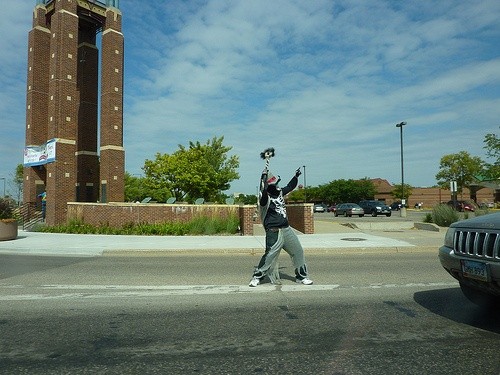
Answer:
[266,171,280,186]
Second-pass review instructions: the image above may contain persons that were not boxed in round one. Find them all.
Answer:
[38,190,46,220]
[415,202,421,209]
[249,169,314,286]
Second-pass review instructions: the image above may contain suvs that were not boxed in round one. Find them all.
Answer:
[439,212,500,310]
[358,200,391,217]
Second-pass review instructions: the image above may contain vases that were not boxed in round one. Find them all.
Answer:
[0,222,18,241]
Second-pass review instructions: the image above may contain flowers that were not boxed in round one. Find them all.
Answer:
[0,197,18,224]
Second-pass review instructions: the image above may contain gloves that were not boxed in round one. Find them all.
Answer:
[295,167,302,177]
[263,169,268,174]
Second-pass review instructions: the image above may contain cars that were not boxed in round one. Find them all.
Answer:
[334,203,365,217]
[314,204,336,212]
[390,201,409,211]
[456,204,474,213]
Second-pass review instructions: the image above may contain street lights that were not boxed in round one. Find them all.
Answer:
[395,122,407,217]
[0,177,6,197]
[303,166,306,203]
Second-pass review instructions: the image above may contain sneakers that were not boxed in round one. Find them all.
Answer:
[249,279,260,287]
[295,278,313,285]
